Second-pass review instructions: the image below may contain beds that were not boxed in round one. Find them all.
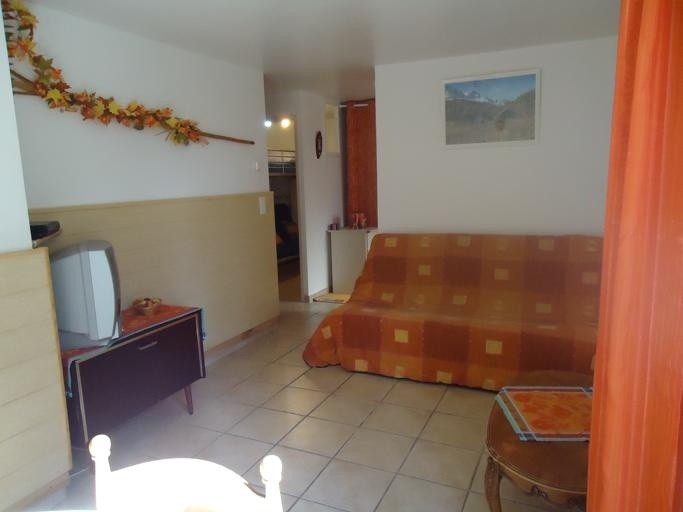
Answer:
[264,148,301,267]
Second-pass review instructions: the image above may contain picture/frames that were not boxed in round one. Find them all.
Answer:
[441,66,544,150]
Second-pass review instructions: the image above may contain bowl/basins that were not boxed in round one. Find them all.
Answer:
[132,298,162,317]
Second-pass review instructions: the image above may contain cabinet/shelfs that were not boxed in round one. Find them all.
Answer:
[48,302,208,458]
[327,228,377,294]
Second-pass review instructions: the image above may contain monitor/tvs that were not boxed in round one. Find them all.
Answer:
[48,239,120,351]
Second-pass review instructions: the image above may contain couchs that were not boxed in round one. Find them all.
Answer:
[304,231,603,393]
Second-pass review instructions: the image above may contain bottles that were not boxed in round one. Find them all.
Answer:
[351,211,366,230]
[330,224,336,230]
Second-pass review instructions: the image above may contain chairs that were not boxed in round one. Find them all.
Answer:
[86,431,286,511]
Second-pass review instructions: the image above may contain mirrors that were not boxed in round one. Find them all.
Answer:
[322,102,342,157]
